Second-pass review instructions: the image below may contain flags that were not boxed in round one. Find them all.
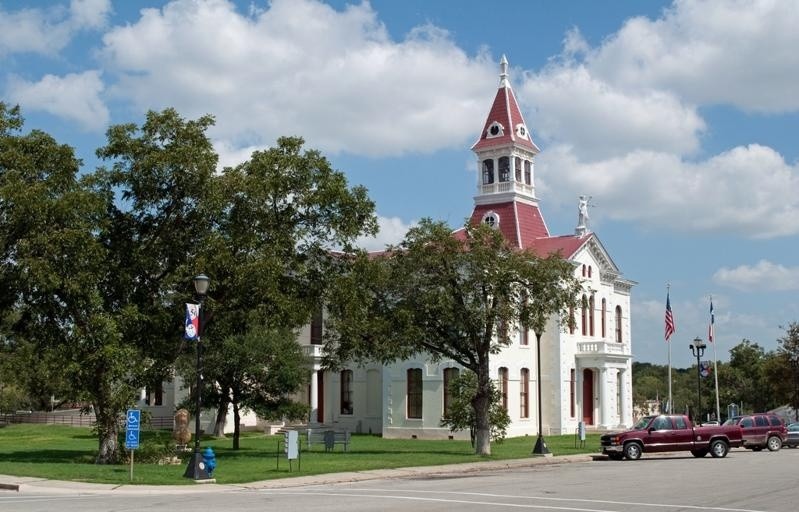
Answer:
[663,290,676,341]
[707,302,715,343]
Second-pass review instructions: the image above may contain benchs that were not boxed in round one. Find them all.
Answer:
[296,429,350,453]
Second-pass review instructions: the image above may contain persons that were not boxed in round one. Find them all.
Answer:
[577,193,592,226]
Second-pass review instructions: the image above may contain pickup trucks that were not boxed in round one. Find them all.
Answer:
[601,412,744,462]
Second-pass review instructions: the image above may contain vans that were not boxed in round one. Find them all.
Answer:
[721,414,788,452]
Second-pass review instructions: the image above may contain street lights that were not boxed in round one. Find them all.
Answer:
[689,336,707,423]
[182,266,217,484]
[530,323,553,458]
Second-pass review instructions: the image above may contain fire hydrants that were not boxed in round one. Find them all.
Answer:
[202,446,216,478]
